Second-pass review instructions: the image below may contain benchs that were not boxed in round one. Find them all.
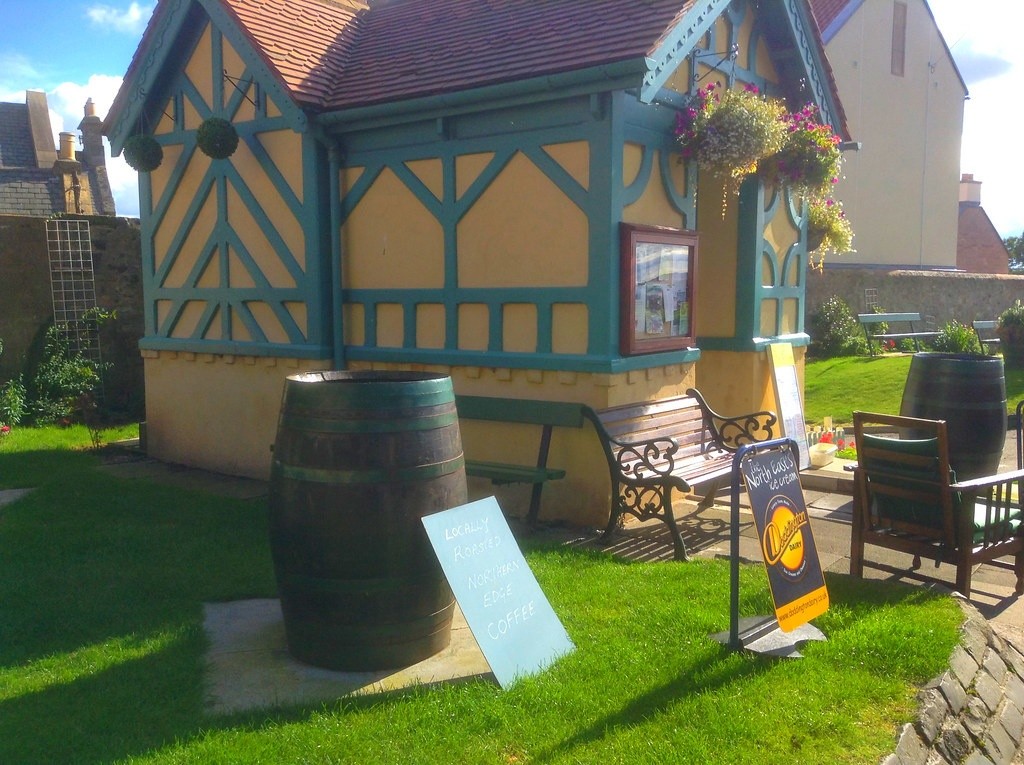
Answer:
[455,391,584,538]
[972,321,1000,354]
[858,313,944,357]
[583,388,777,563]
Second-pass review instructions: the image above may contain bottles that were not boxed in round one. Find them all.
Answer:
[807,427,846,452]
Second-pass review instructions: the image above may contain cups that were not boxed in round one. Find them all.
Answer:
[824,417,832,430]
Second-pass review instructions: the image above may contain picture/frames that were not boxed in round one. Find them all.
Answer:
[618,221,699,356]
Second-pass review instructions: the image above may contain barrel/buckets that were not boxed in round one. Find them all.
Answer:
[898,351,1008,496]
[269,368,468,672]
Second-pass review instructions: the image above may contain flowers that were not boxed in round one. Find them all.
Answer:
[759,103,842,200]
[670,79,788,219]
[801,191,857,276]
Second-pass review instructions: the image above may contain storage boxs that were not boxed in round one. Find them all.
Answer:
[809,441,838,466]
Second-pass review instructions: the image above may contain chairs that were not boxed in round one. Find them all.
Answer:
[849,410,1024,606]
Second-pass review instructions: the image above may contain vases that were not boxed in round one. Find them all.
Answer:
[807,228,827,251]
